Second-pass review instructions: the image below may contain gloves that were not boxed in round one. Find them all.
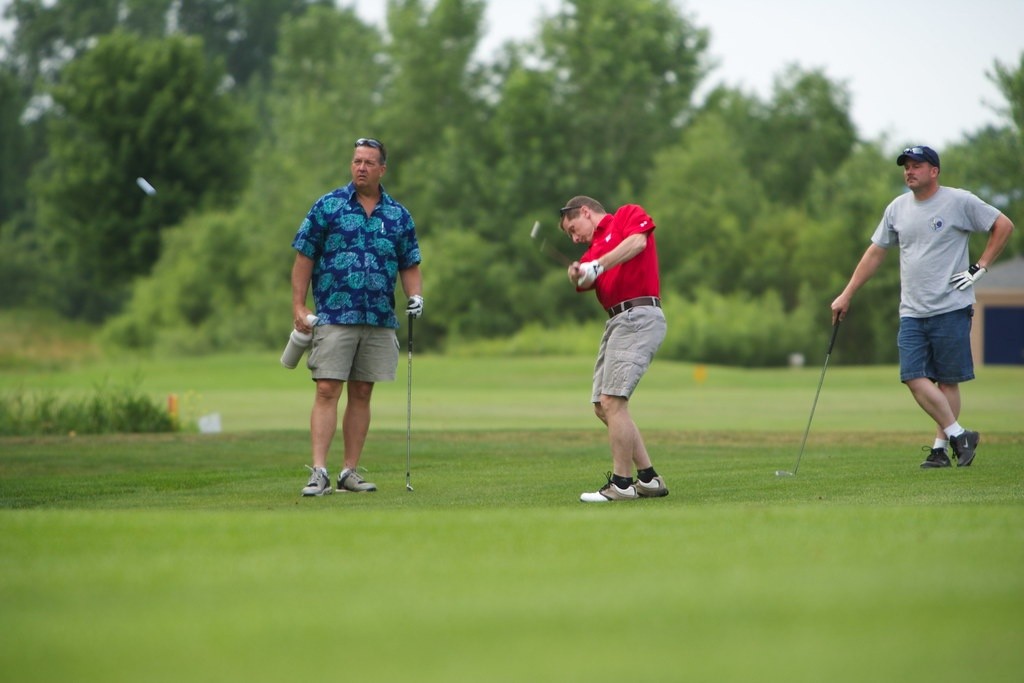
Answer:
[578,260,605,290]
[949,263,987,291]
[406,294,424,317]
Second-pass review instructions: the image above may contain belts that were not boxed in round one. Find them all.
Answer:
[608,298,661,318]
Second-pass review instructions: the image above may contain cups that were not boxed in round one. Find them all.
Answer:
[280,329,314,369]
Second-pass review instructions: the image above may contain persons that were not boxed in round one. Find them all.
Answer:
[290,138,425,498]
[559,196,671,502]
[830,144,1014,468]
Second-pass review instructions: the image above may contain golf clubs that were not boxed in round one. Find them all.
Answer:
[529,219,580,271]
[774,312,842,478]
[405,312,415,494]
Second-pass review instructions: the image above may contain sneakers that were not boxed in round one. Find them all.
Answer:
[918,446,951,468]
[302,465,332,497]
[949,429,980,466]
[335,466,375,492]
[580,471,638,502]
[634,475,669,497]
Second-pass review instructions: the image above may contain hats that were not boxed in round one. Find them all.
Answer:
[897,146,939,167]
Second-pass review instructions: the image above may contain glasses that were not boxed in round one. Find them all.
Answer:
[355,138,383,149]
[560,206,581,218]
[903,148,937,163]
